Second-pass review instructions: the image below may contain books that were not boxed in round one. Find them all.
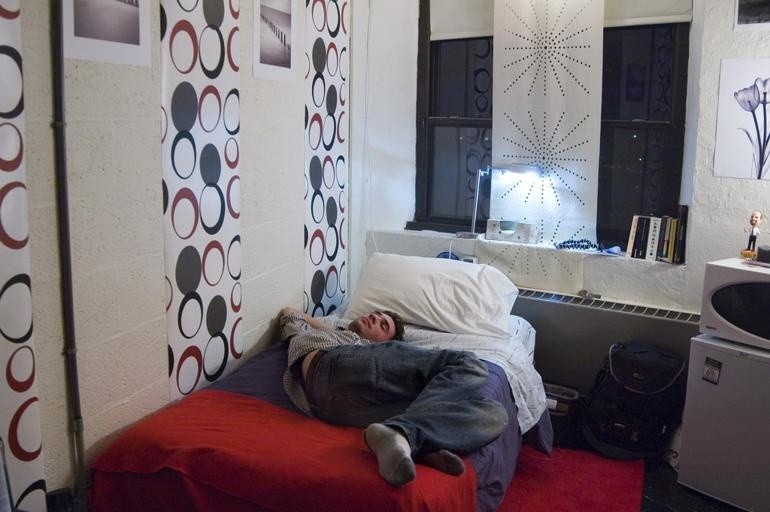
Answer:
[625,205,688,265]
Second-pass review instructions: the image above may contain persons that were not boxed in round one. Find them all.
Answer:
[744,211,762,251]
[279,306,509,485]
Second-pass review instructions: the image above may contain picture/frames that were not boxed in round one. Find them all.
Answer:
[733,0,770,31]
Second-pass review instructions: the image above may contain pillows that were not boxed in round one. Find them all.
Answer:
[340,249,519,337]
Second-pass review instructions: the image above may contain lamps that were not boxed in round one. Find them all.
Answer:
[455,162,542,239]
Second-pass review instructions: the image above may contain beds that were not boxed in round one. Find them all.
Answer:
[93,315,535,511]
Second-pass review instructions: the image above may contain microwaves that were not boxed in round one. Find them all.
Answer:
[699,258,769,354]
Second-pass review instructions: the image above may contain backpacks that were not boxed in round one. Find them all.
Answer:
[573,339,688,462]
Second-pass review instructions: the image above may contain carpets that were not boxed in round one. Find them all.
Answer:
[494,439,648,510]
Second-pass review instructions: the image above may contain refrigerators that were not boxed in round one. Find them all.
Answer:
[675,333,770,511]
